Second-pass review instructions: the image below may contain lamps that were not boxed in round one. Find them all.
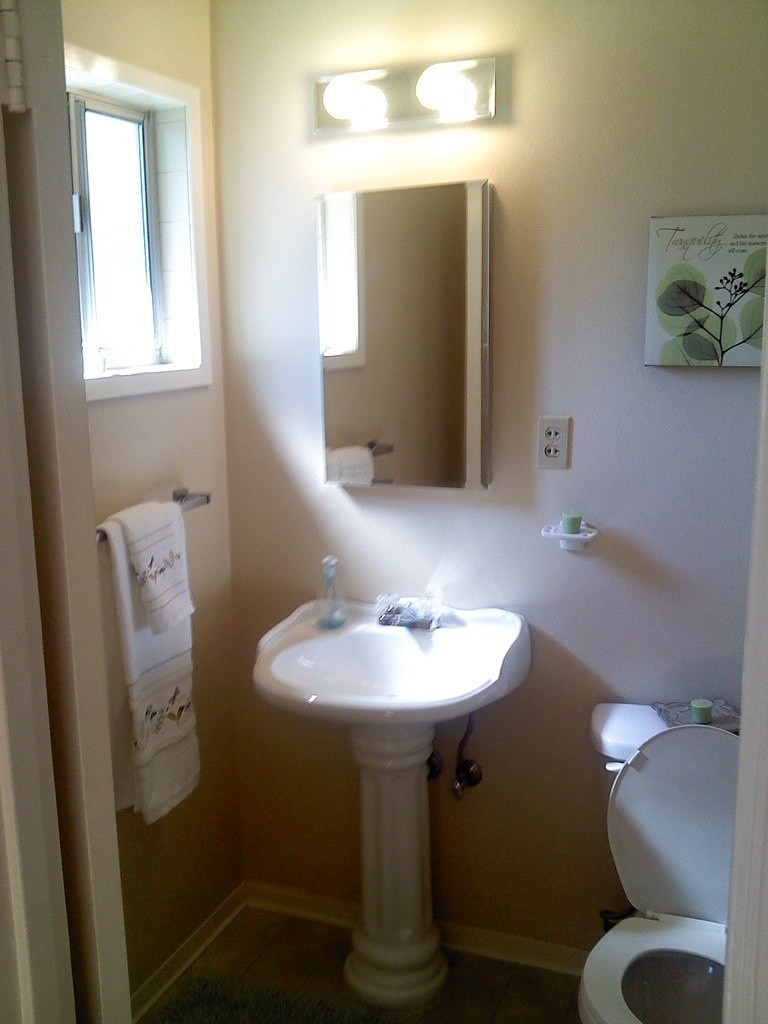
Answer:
[313,57,497,136]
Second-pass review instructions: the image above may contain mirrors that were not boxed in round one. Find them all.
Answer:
[318,178,489,495]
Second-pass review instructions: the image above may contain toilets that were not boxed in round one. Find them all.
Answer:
[574,698,743,1024]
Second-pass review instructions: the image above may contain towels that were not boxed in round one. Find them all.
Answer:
[95,498,204,829]
[325,444,376,486]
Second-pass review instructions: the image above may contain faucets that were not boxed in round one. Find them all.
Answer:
[379,603,416,627]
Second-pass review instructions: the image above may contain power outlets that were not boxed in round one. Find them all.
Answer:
[536,416,572,470]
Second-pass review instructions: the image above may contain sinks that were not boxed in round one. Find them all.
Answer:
[252,594,532,724]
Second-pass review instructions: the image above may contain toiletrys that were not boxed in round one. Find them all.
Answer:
[313,553,347,630]
